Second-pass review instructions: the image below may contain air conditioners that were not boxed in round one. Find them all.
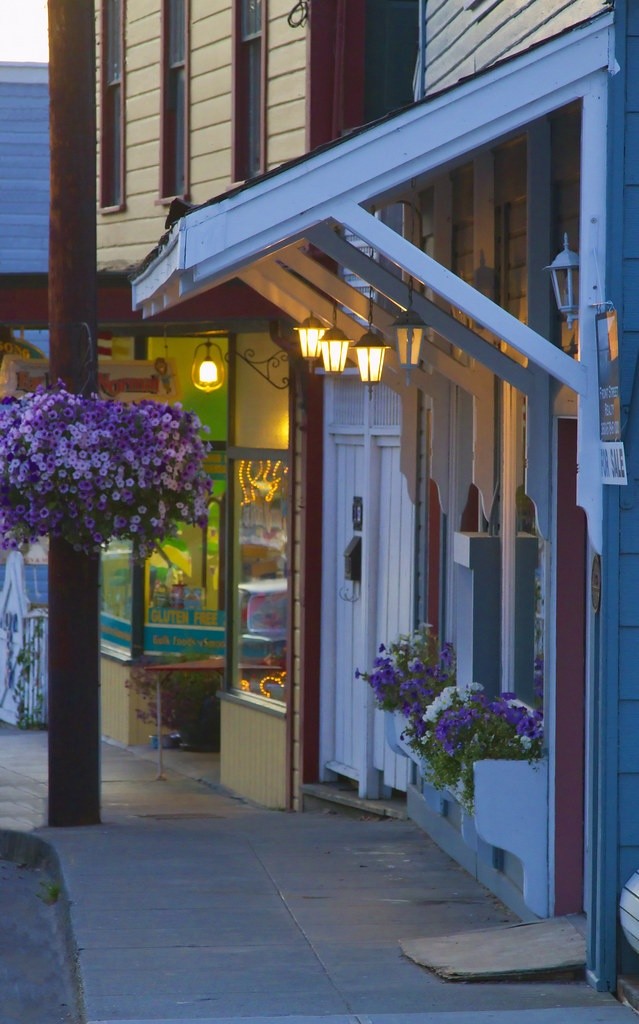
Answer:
[191,338,224,393]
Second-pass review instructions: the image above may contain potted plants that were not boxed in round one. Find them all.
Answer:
[126,655,244,750]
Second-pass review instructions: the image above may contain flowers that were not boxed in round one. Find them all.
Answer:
[0,378,215,564]
[533,653,544,709]
[354,623,547,818]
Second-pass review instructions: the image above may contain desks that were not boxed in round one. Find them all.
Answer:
[143,657,285,782]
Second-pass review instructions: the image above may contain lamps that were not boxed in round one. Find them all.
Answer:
[543,232,579,330]
[349,205,392,401]
[319,223,355,379]
[378,178,432,388]
[293,243,331,373]
